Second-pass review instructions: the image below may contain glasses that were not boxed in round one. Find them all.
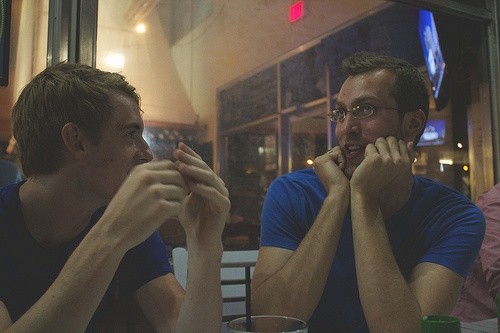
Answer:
[328,101,413,123]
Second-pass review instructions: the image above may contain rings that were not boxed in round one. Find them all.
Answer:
[327,148,333,153]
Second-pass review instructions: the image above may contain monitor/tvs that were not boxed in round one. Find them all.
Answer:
[417,6,448,102]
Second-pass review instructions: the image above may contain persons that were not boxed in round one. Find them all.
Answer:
[451,181,500,323]
[251,51,486,333]
[0,62,231,333]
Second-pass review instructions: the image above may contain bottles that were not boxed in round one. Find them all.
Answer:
[422,315,462,333]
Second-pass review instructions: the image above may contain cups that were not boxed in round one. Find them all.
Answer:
[227,316,310,333]
